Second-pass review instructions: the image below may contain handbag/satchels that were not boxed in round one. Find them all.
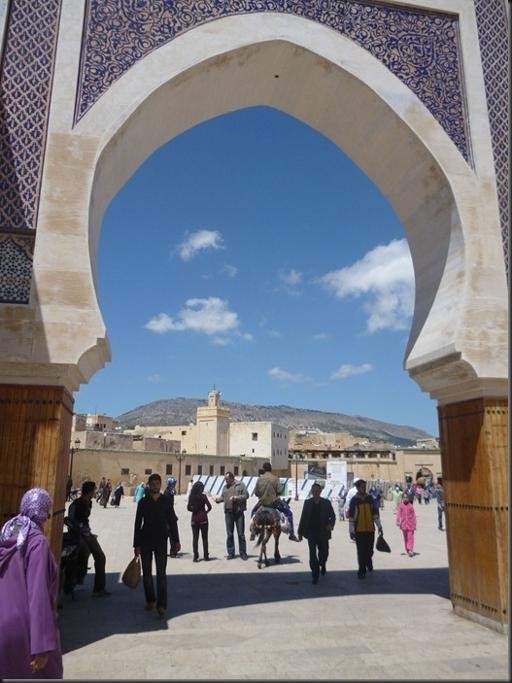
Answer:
[122,555,141,589]
[377,529,391,554]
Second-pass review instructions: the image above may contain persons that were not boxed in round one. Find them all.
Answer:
[164,478,181,558]
[96,476,105,501]
[100,479,112,509]
[397,492,418,557]
[404,482,439,504]
[250,462,301,542]
[349,480,383,579]
[133,474,173,618]
[135,481,145,502]
[186,480,193,496]
[392,487,403,515]
[298,483,336,585]
[437,483,447,530]
[66,475,73,501]
[187,482,211,561]
[369,484,385,508]
[67,481,112,598]
[338,485,347,521]
[0,488,63,679]
[214,472,249,560]
[113,482,124,507]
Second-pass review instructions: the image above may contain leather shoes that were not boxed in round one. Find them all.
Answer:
[358,567,366,579]
[227,554,236,560]
[367,562,373,573]
[240,550,247,560]
[312,573,319,585]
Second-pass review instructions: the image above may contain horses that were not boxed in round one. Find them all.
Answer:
[254,498,289,569]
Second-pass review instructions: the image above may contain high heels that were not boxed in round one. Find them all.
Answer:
[203,554,208,561]
[191,554,200,562]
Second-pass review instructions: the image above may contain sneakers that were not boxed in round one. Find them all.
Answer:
[156,605,165,618]
[145,602,155,610]
[93,588,112,599]
[321,566,326,576]
